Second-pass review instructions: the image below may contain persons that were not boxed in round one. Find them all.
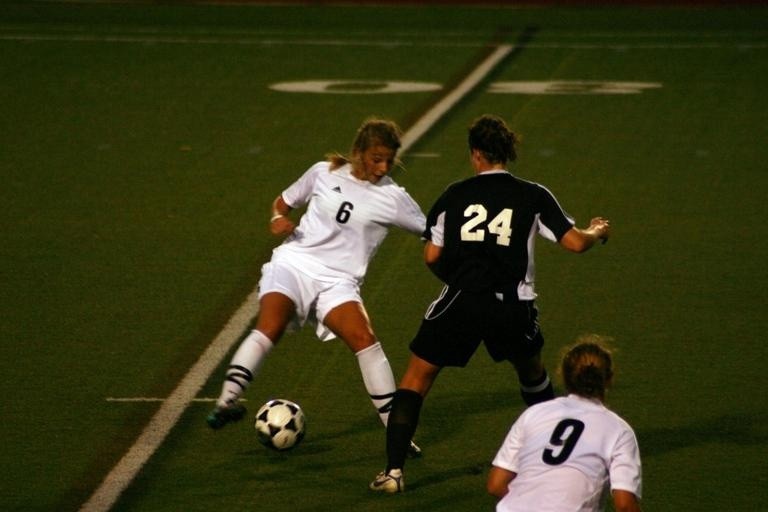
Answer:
[206,116,430,459]
[369,113,612,496]
[484,333,649,512]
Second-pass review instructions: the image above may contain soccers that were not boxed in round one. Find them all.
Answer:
[253,399,304,449]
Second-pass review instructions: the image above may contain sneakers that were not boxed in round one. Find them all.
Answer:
[410,443,422,456]
[370,470,405,493]
[208,400,246,429]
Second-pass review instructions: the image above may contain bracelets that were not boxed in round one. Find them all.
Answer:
[269,213,285,223]
